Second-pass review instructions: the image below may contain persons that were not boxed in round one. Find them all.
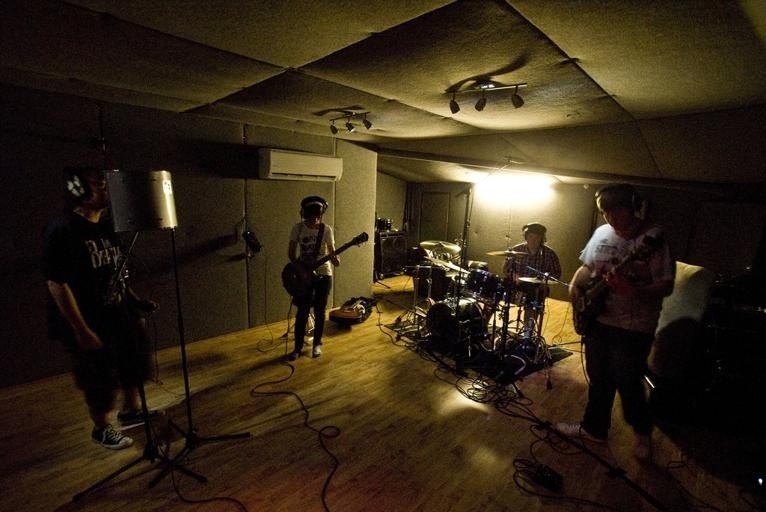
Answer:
[554,182,673,459]
[288,196,340,361]
[39,162,166,450]
[482,222,562,350]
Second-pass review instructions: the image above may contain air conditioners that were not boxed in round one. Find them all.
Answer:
[258,148,343,182]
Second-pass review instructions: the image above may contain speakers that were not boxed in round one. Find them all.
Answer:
[378,234,408,275]
[675,308,766,437]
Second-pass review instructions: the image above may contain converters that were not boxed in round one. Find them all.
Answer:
[536,464,562,484]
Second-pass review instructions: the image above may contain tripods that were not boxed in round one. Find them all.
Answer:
[394,277,552,392]
[149,231,251,488]
[72,268,208,504]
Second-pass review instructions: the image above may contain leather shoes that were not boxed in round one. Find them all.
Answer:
[313,345,321,357]
[289,348,303,360]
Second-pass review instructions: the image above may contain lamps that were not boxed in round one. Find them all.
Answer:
[448,81,528,113]
[330,111,371,134]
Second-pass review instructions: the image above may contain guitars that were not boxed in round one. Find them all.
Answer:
[329,300,366,322]
[573,215,656,335]
[282,231,368,295]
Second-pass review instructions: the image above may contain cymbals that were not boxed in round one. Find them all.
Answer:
[518,276,558,285]
[486,250,528,256]
[420,240,461,255]
[424,256,471,274]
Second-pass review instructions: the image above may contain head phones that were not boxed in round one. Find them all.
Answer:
[595,183,643,213]
[64,173,86,200]
[300,201,328,217]
[522,225,545,246]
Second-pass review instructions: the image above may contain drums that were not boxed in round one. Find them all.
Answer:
[466,268,499,300]
[426,294,488,348]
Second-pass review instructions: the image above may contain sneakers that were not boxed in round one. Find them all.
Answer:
[634,434,651,459]
[91,423,133,450]
[556,422,609,445]
[117,408,166,430]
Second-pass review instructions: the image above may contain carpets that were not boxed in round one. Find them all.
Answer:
[384,322,573,386]
